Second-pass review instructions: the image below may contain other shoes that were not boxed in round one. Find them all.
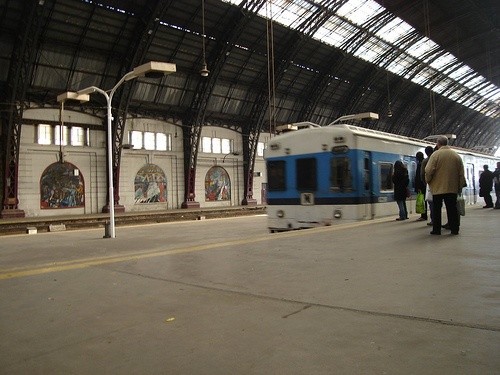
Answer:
[395,216,459,235]
[483,201,500,209]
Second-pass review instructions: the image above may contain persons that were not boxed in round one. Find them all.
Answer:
[391,160,410,220]
[411,152,428,221]
[426,135,466,235]
[491,162,500,209]
[479,164,495,208]
[421,146,436,225]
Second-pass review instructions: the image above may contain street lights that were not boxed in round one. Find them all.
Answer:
[55,59,178,238]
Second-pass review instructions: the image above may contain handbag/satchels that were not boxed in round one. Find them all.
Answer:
[415,189,427,215]
[456,192,466,217]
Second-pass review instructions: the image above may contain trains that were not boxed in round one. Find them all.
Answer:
[261,122,500,235]
